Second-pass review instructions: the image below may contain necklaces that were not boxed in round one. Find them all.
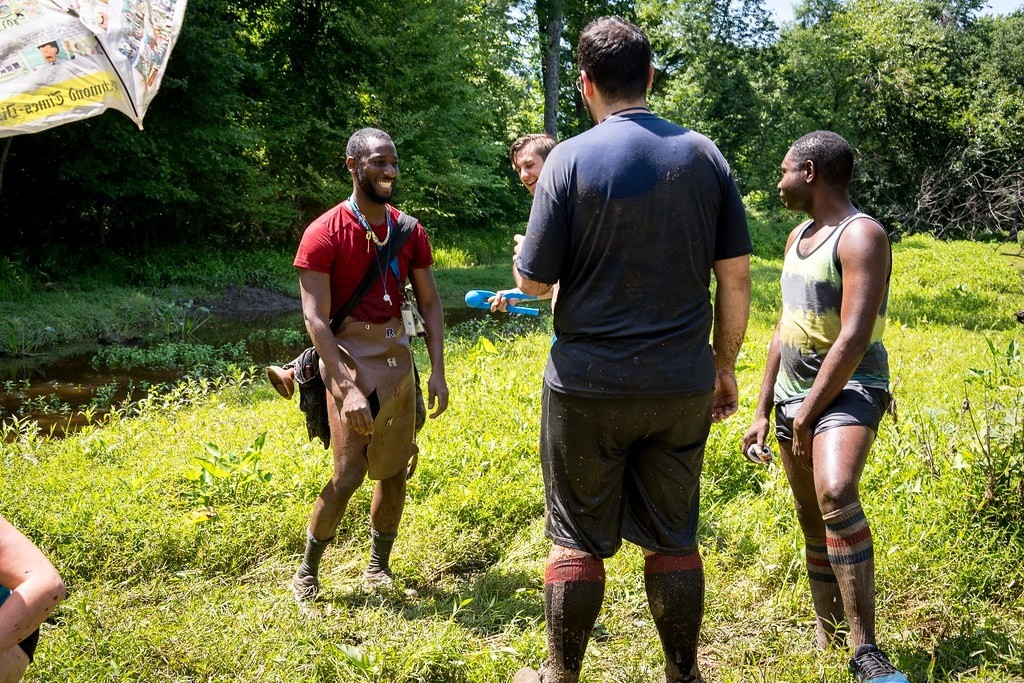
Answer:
[348,196,391,246]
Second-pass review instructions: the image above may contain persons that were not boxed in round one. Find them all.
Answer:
[0,515,66,683]
[291,127,449,620]
[511,17,751,683]
[742,131,912,683]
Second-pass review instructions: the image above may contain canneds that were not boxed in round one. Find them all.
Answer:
[747,444,772,464]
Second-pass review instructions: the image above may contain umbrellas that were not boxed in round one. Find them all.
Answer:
[0,0,188,139]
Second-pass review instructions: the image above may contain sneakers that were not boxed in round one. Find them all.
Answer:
[363,567,419,597]
[848,643,907,683]
[291,566,323,618]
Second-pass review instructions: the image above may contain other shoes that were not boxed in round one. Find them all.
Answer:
[512,666,550,683]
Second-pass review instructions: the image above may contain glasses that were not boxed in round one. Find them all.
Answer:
[576,76,583,91]
